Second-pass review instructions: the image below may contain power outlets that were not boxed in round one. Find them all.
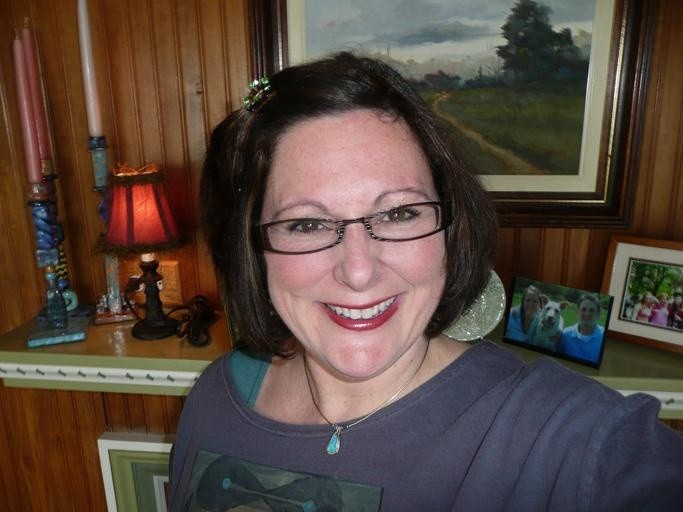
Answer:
[123,260,183,305]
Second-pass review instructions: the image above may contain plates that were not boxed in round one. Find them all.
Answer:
[440,269,505,341]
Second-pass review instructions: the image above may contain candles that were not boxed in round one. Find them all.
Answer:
[76,0,107,147]
[23,18,54,176]
[13,29,47,199]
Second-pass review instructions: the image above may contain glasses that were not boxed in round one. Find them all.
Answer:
[253,202,448,255]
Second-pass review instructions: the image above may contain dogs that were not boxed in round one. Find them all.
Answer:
[536,294,569,352]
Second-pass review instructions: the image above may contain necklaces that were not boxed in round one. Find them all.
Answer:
[302,337,430,455]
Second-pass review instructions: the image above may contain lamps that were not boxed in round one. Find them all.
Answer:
[104,165,191,340]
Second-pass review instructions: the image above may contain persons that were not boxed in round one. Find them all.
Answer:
[624,291,683,330]
[504,284,543,346]
[557,294,606,364]
[167,50,683,512]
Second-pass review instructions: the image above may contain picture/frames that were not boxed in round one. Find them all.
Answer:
[247,0,659,230]
[97,431,178,512]
[599,235,683,352]
